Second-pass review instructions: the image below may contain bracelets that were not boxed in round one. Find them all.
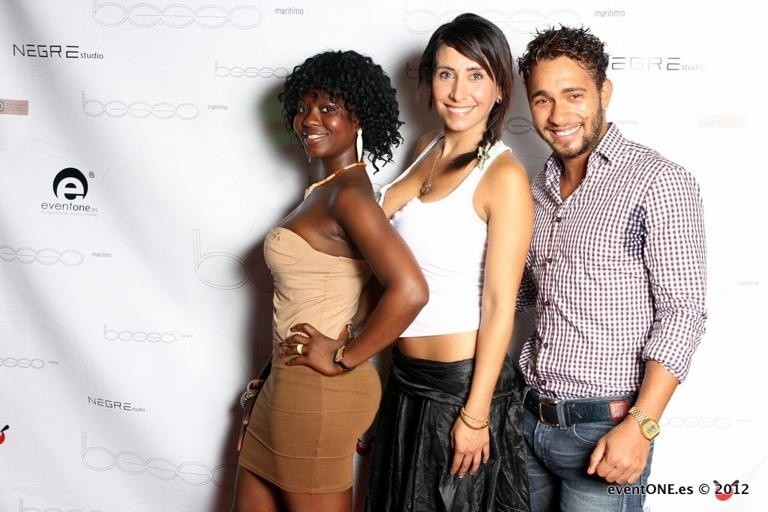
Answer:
[240,390,257,410]
[246,378,264,391]
[461,404,491,422]
[460,409,490,430]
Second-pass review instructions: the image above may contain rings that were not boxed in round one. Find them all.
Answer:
[296,344,304,355]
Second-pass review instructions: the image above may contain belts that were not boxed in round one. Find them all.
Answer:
[518,387,636,427]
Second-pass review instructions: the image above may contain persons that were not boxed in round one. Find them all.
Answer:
[231,48,429,512]
[361,11,530,512]
[491,22,706,511]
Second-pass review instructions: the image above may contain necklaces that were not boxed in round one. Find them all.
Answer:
[419,140,460,195]
[304,160,366,198]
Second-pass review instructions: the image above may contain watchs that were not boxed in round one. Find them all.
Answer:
[626,406,660,442]
[333,343,355,373]
